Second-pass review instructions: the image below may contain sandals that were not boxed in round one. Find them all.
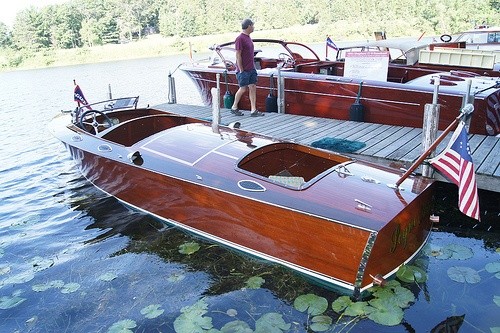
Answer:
[230,107,244,116]
[250,110,266,116]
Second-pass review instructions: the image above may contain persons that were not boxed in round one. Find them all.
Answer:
[230,18,265,117]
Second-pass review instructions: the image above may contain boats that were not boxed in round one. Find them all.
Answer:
[178,17,500,136]
[46,80,475,300]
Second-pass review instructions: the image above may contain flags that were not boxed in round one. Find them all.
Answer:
[326,36,340,52]
[74,84,93,111]
[424,122,482,229]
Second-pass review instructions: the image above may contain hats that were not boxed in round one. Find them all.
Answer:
[242,19,254,29]
